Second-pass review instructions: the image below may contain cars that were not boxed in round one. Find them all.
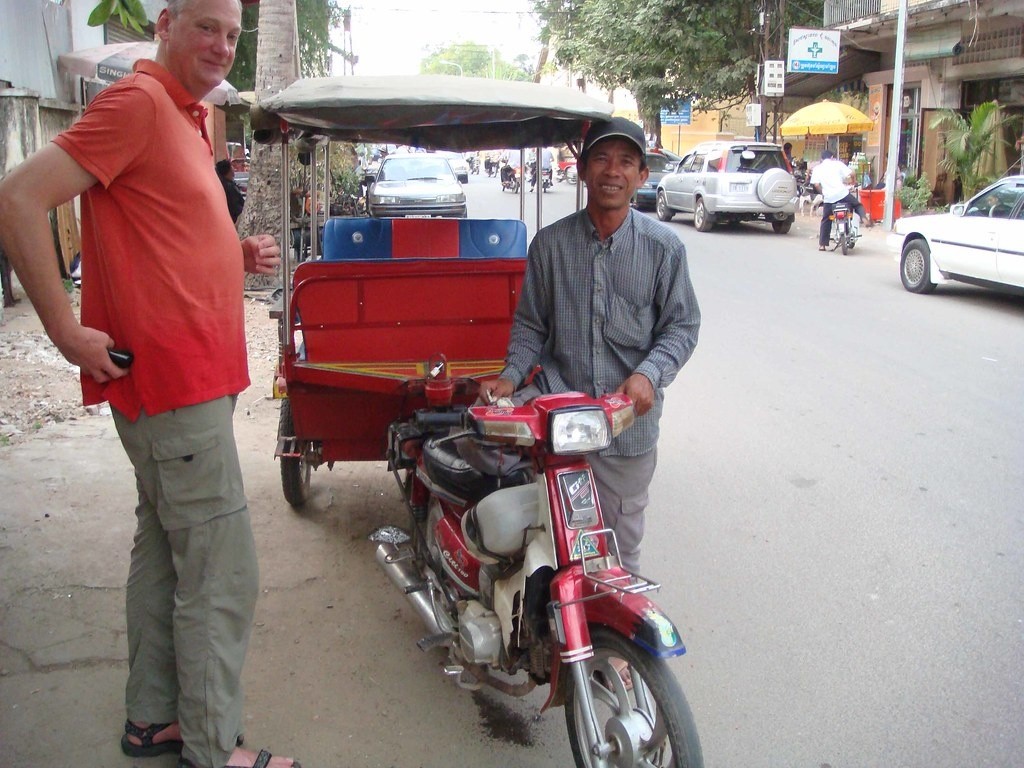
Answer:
[449,158,469,183]
[892,174,1024,294]
[364,154,468,220]
[229,157,252,197]
[631,148,696,212]
[557,144,582,185]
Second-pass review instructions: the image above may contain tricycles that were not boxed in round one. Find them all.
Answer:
[249,74,619,507]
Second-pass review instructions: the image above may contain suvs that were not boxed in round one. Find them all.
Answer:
[656,140,800,235]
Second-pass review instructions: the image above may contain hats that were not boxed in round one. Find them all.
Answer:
[581,117,647,169]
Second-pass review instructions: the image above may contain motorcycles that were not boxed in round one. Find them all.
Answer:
[468,155,552,194]
[364,360,710,768]
[824,184,864,254]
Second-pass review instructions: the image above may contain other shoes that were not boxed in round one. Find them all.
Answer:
[863,218,872,228]
[819,246,826,251]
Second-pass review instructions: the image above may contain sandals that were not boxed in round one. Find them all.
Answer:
[121,716,245,758]
[178,742,301,767]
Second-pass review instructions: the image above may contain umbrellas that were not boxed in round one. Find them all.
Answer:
[57,41,241,107]
[779,98,874,160]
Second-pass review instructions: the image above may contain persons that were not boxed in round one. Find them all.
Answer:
[368,142,555,192]
[0,0,302,768]
[478,117,701,695]
[985,195,1004,207]
[783,141,902,253]
[215,157,245,224]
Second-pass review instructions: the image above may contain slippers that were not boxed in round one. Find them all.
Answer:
[601,654,633,692]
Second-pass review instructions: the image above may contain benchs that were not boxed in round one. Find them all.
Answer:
[321,216,527,260]
[289,257,527,363]
